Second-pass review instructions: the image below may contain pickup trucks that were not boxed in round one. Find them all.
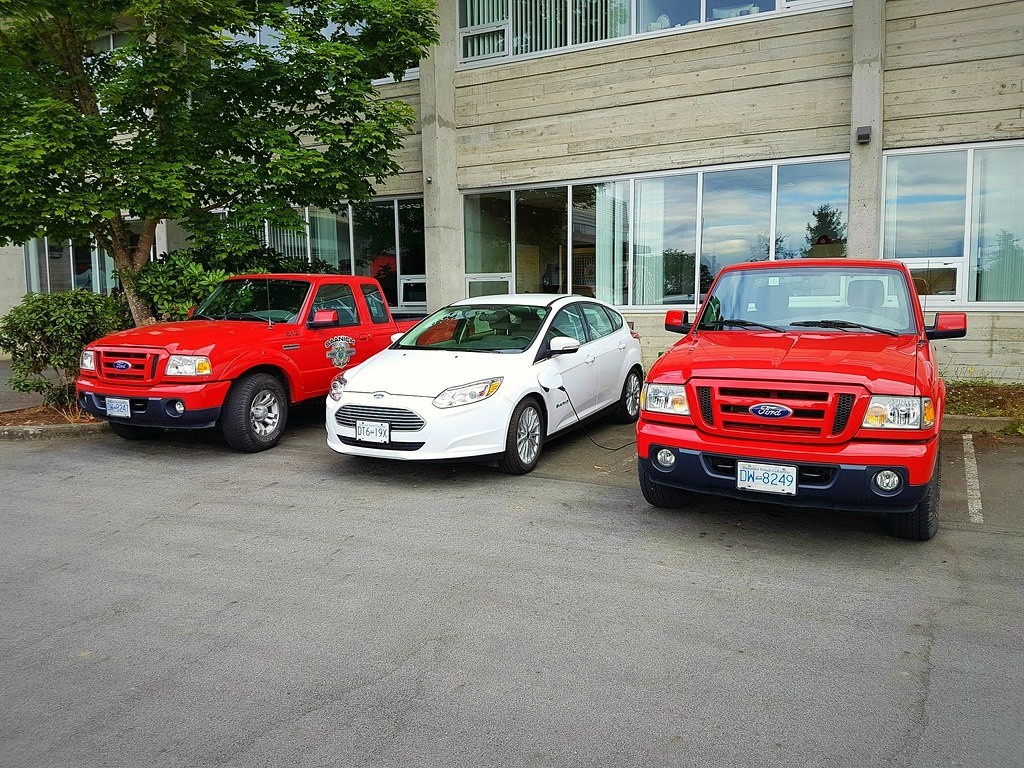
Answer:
[75,272,476,453]
[637,258,966,541]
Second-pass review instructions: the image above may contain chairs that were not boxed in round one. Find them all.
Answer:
[486,312,512,334]
[755,285,787,318]
[846,279,886,315]
[521,316,540,335]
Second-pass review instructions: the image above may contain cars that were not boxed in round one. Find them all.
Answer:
[325,295,645,475]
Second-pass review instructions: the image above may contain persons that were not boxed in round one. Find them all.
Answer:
[543,264,559,293]
